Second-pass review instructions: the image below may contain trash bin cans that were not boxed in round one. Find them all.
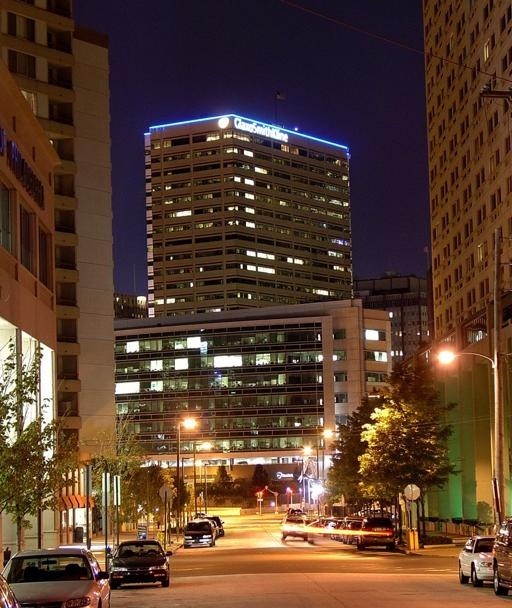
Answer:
[75,527,83,543]
[407,528,419,550]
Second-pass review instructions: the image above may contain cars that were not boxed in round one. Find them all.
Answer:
[454,535,495,588]
[3,546,114,608]
[1,573,23,608]
[181,511,226,550]
[105,539,175,587]
[282,508,399,550]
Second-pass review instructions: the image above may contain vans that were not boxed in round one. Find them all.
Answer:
[491,517,512,597]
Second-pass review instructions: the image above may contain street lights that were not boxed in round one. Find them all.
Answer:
[192,442,213,519]
[176,415,203,543]
[435,346,507,525]
[181,454,196,525]
[203,462,210,513]
[287,428,332,508]
[196,459,202,513]
